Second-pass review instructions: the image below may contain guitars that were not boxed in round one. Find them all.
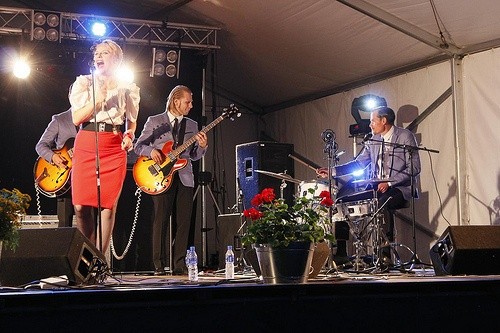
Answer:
[131,102,242,197]
[32,135,76,198]
[252,169,300,185]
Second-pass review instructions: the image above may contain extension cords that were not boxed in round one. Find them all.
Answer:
[40,276,70,288]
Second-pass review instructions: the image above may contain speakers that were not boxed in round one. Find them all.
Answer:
[234,142,294,214]
[429,226,500,276]
[101,167,174,275]
[0,227,109,286]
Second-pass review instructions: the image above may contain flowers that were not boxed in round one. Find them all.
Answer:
[241,188,337,251]
[0,188,31,252]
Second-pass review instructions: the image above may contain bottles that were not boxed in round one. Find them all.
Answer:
[185,247,198,281]
[225,246,234,279]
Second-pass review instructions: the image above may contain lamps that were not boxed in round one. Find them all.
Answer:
[150,47,180,80]
[31,10,63,44]
[351,94,387,132]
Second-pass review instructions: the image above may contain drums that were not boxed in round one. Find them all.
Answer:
[286,213,331,250]
[346,197,382,217]
[295,176,341,205]
[304,201,348,225]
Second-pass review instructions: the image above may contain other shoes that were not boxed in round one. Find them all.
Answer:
[334,256,352,266]
[172,271,188,276]
[154,269,165,276]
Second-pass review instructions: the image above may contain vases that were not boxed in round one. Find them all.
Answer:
[255,240,316,285]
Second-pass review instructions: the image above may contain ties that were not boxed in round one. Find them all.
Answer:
[379,138,383,180]
[172,118,179,150]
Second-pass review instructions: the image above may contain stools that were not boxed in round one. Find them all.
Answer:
[376,200,410,272]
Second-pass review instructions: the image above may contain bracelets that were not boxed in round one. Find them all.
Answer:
[123,130,135,141]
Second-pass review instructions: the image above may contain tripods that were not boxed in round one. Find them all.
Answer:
[327,138,440,274]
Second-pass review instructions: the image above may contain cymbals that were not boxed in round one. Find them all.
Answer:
[349,178,399,187]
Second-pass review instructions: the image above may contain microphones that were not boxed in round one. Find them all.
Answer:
[359,132,373,144]
[100,79,106,111]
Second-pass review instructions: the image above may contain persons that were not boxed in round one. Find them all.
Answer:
[134,85,208,276]
[316,106,422,274]
[36,84,81,227]
[70,39,141,254]
